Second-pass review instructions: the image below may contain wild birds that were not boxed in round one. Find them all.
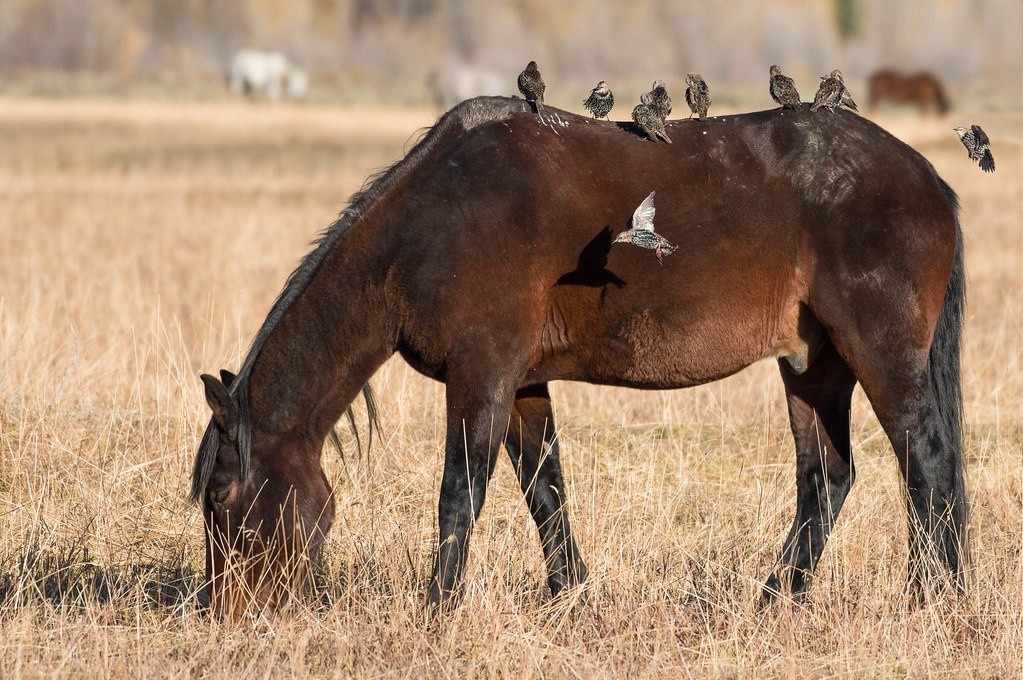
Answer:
[809,67,859,118]
[517,60,545,115]
[631,79,674,145]
[953,123,997,174]
[585,80,616,121]
[610,191,680,267]
[769,65,805,114]
[684,72,712,121]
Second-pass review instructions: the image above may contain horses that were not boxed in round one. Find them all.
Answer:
[187,94,973,639]
